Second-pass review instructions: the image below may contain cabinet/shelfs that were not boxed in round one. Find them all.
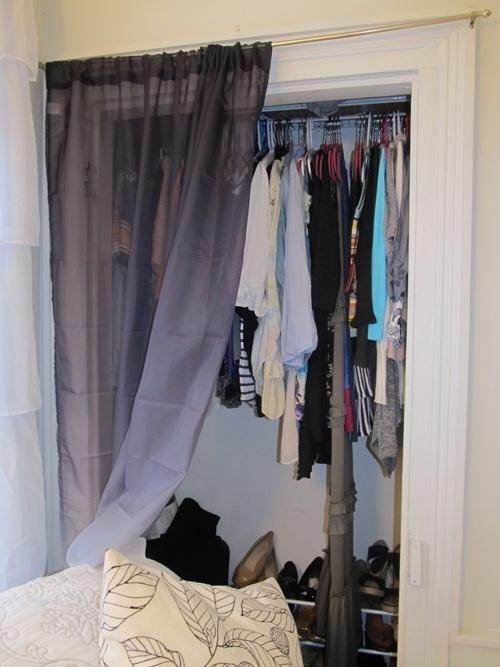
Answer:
[280,596,400,660]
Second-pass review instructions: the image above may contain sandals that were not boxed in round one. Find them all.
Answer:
[382,543,399,612]
[360,537,389,598]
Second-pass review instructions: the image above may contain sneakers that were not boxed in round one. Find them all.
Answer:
[350,554,367,597]
[287,602,398,667]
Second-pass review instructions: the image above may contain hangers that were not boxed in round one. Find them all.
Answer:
[252,104,409,184]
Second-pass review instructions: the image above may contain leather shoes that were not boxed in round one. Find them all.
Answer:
[280,559,297,610]
[231,529,274,587]
[258,550,279,589]
[299,554,323,601]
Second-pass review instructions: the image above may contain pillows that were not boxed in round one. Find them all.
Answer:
[0,563,104,665]
[96,546,303,666]
